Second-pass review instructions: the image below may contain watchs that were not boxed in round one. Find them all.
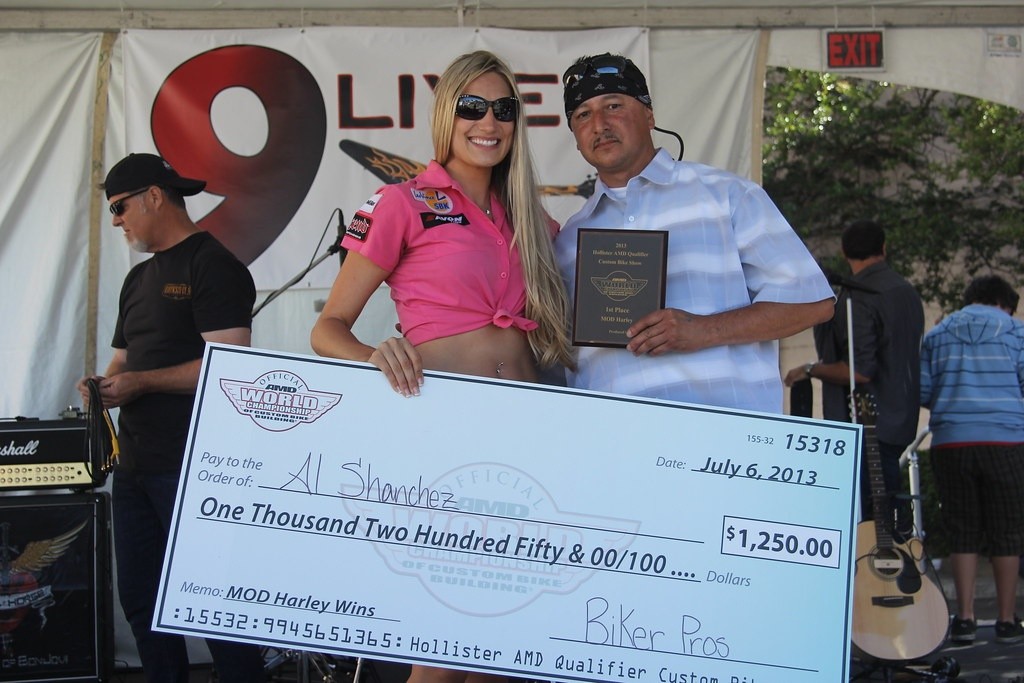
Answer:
[804,363,816,376]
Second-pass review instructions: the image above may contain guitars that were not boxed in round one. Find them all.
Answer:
[839,391,951,661]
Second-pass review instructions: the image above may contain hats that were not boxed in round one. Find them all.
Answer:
[105,153,207,196]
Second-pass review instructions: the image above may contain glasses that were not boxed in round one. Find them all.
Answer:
[562,55,639,90]
[109,184,162,217]
[455,95,520,121]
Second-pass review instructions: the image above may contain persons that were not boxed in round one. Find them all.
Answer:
[553,50,839,416]
[785,218,925,539]
[918,274,1024,644]
[309,49,561,683]
[76,152,272,683]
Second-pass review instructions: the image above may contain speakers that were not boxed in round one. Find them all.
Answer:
[0,492,114,683]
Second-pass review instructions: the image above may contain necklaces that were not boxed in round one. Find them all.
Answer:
[485,209,491,215]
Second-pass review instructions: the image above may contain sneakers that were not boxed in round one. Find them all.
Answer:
[995,614,1024,643]
[951,613,976,642]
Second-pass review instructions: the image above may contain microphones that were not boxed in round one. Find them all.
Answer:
[338,210,348,267]
[826,275,880,295]
